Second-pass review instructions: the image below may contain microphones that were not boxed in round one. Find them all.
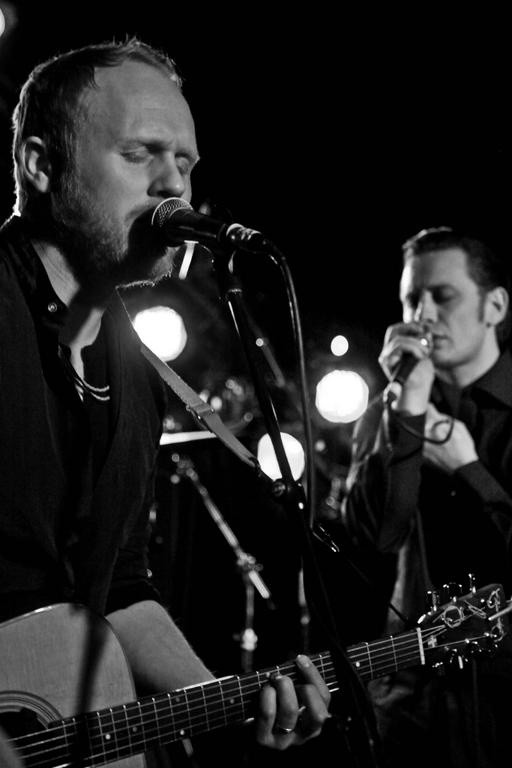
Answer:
[149,196,266,254]
[383,325,434,404]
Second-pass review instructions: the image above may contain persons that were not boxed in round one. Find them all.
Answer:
[340,228,511,766]
[0,42,334,768]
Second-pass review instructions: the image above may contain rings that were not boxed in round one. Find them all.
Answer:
[275,722,293,735]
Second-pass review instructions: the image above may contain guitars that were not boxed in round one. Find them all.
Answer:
[0,570,510,768]
